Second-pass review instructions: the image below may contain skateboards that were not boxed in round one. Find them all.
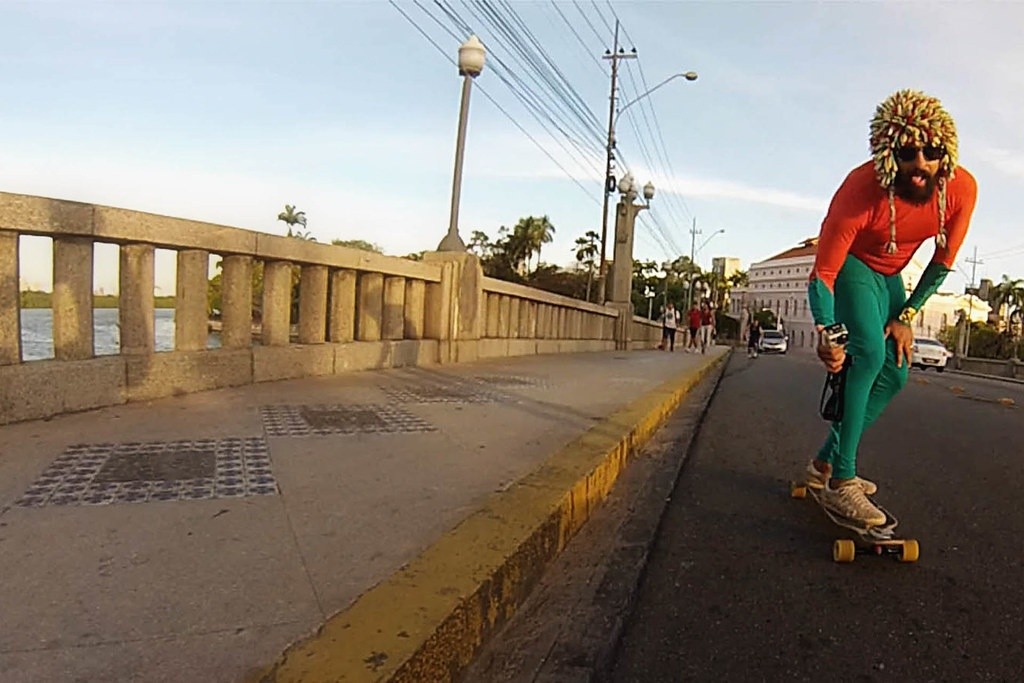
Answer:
[790,480,919,563]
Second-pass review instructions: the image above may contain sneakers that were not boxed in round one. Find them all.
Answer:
[821,477,887,526]
[804,460,877,494]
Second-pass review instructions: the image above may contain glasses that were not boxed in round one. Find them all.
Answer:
[895,145,944,160]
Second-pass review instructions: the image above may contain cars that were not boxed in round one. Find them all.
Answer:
[758,330,788,354]
[908,336,948,373]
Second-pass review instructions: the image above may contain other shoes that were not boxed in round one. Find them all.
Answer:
[702,347,705,354]
[659,345,664,350]
[694,348,698,353]
[748,353,752,358]
[671,346,673,351]
[753,352,758,357]
[685,347,690,353]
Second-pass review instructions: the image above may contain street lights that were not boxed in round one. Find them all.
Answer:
[688,228,725,309]
[438,34,485,252]
[762,307,782,331]
[596,71,697,304]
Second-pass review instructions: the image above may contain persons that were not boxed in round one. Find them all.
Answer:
[741,305,748,338]
[808,89,977,524]
[745,319,763,358]
[685,301,716,354]
[659,303,680,352]
